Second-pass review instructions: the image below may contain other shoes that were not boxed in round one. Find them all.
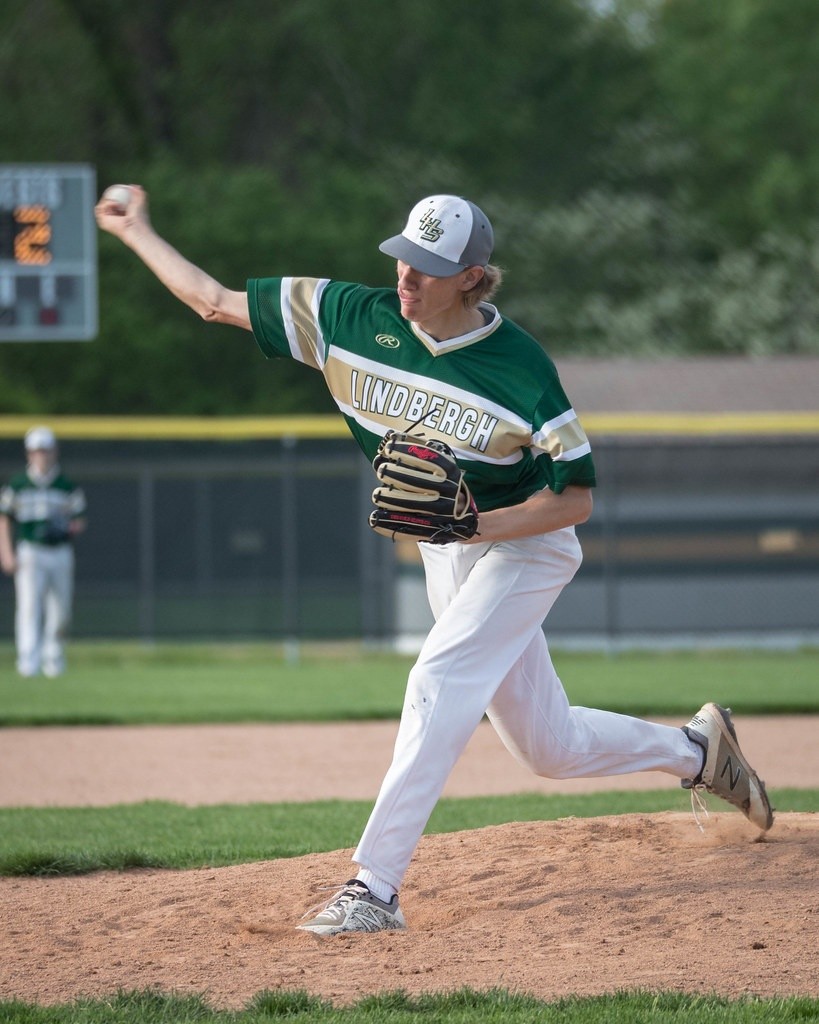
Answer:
[17,647,41,678]
[43,646,66,677]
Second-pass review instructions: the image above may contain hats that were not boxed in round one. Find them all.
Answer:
[23,425,58,451]
[377,193,497,279]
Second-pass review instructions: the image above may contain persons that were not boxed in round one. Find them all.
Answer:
[92,183,777,939]
[0,426,87,679]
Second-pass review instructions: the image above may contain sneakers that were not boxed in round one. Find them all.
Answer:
[292,877,408,937]
[679,701,776,835]
